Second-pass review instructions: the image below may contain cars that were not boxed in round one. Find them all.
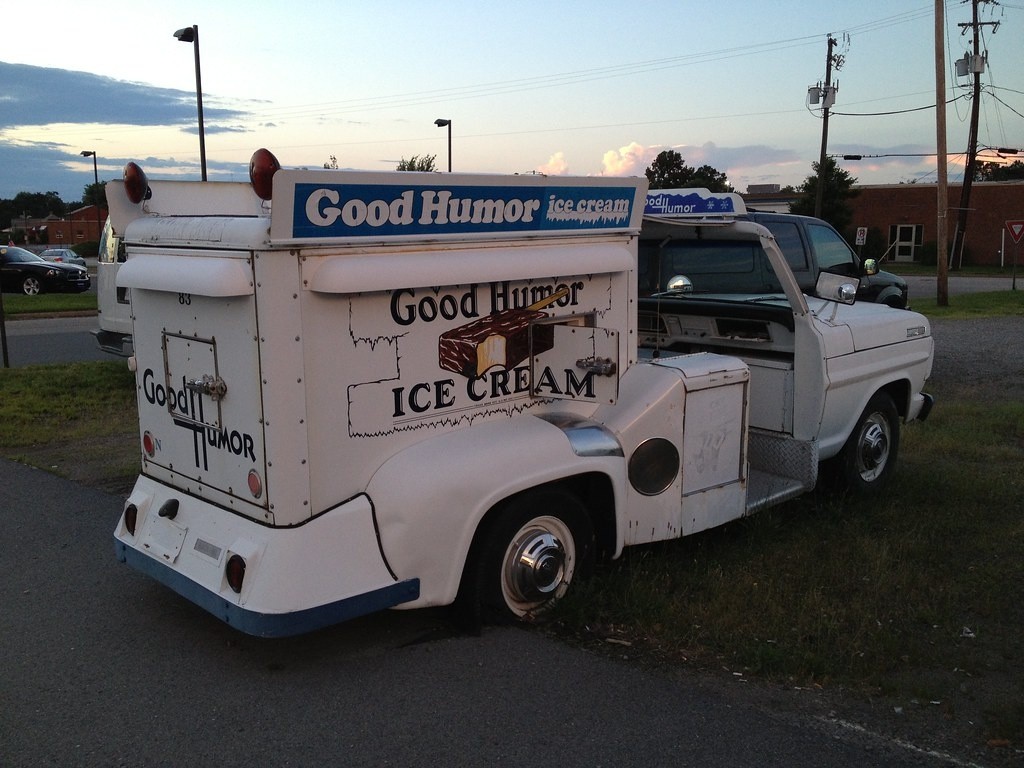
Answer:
[0,246,91,296]
[38,248,87,267]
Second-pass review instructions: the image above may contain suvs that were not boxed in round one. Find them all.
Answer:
[639,212,911,309]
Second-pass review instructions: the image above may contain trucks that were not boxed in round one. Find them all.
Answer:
[103,143,935,638]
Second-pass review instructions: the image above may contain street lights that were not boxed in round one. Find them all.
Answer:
[173,24,207,181]
[435,118,452,173]
[81,150,102,238]
